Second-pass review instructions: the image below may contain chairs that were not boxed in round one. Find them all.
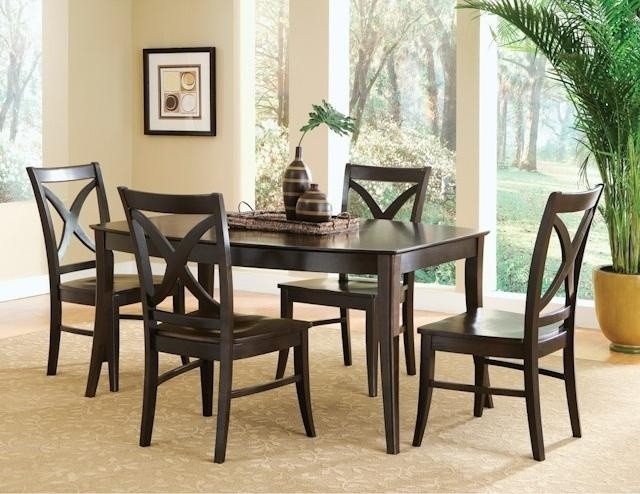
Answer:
[117,185,316,464]
[26,162,189,392]
[413,184,605,460]
[275,164,431,397]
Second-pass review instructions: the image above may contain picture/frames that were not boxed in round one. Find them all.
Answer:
[143,46,215,136]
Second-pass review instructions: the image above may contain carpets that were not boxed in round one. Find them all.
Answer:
[0,306,640,494]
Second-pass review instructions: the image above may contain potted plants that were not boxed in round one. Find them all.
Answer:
[454,0,640,353]
[283,100,359,219]
